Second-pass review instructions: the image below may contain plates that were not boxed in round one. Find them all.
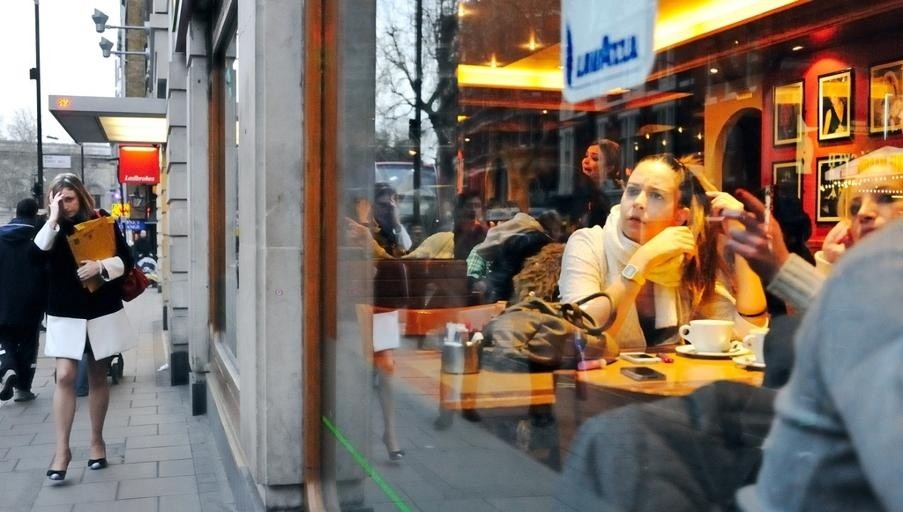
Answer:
[729,355,767,370]
[671,342,748,358]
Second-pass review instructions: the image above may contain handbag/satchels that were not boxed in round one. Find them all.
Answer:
[122,268,149,301]
[483,293,616,371]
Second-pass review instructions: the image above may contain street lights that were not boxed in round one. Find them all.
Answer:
[129,178,149,267]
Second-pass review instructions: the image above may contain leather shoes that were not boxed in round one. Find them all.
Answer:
[47,449,71,480]
[88,441,106,469]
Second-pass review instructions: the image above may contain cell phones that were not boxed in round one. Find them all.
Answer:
[620,367,666,382]
[619,352,661,364]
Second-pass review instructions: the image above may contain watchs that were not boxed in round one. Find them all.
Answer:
[621,263,646,287]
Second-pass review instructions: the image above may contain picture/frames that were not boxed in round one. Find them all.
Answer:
[815,153,854,223]
[771,158,803,208]
[772,78,805,148]
[867,56,902,136]
[817,66,855,143]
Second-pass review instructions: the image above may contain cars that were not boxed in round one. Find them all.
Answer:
[129,232,157,285]
[376,150,624,264]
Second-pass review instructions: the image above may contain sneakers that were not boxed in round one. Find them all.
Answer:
[0,369,17,400]
[13,388,34,400]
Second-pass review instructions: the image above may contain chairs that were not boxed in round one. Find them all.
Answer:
[370,258,480,310]
[552,370,680,476]
[356,299,507,355]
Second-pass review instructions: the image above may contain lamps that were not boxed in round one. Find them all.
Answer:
[92,7,155,60]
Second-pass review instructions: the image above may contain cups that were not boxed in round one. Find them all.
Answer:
[439,340,479,376]
[678,318,734,353]
[743,327,767,361]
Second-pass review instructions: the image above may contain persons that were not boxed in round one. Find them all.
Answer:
[0,198,44,403]
[372,181,413,462]
[734,217,902,511]
[823,79,847,134]
[33,172,135,480]
[779,104,797,139]
[722,144,903,311]
[557,154,770,349]
[466,200,553,303]
[580,139,622,185]
[879,70,902,126]
[433,189,488,429]
